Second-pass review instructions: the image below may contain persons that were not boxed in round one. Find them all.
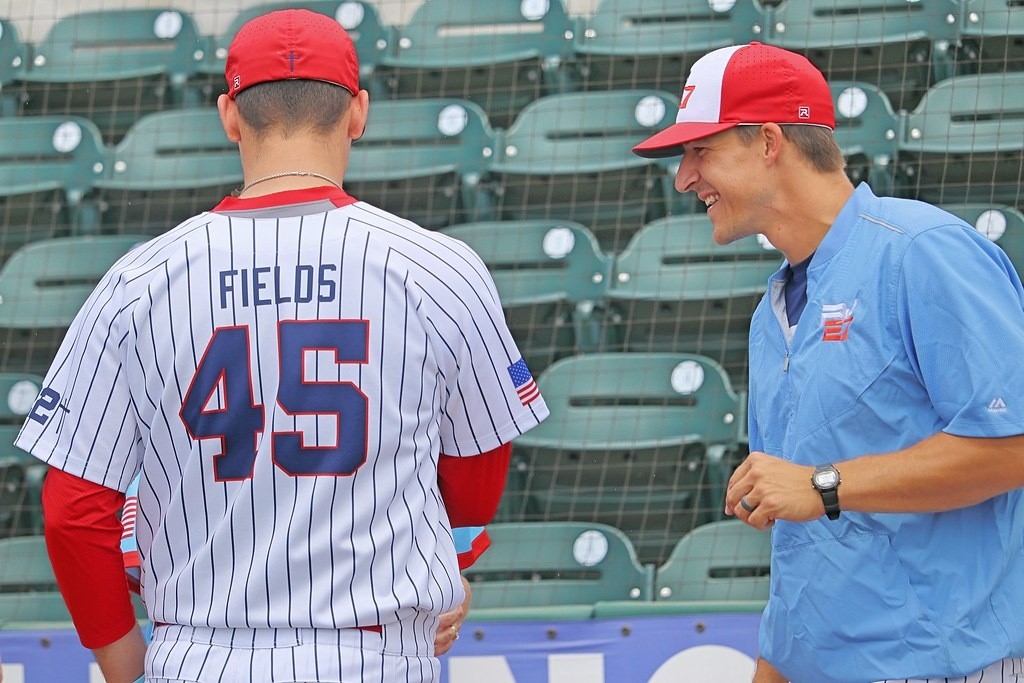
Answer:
[15,9,548,683]
[632,42,1024,683]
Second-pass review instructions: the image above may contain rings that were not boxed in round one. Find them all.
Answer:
[450,624,459,643]
[741,496,756,513]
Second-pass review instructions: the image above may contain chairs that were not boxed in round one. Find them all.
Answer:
[1,0,1024,633]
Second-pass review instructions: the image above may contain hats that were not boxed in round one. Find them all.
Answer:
[225,8,361,98]
[631,40,838,159]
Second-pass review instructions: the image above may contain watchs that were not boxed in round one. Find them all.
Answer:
[811,463,842,522]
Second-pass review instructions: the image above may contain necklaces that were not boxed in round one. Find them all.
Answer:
[241,170,344,193]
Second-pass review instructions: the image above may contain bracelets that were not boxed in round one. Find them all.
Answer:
[133,672,147,683]
